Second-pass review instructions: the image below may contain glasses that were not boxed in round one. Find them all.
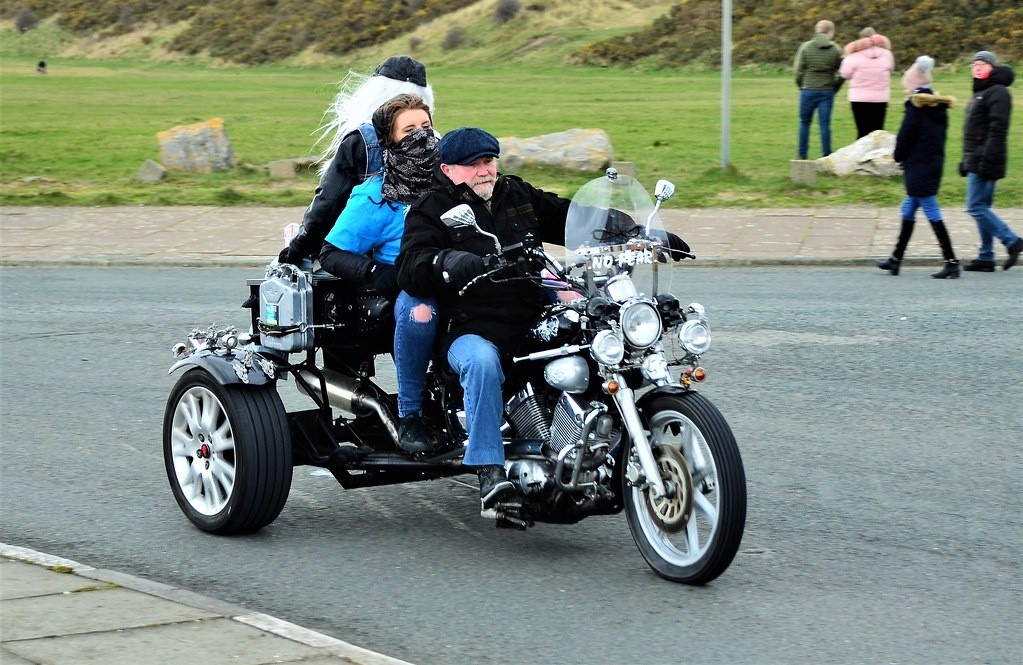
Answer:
[970,63,990,70]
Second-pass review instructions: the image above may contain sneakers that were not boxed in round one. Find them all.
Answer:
[478,464,516,509]
[398,411,433,452]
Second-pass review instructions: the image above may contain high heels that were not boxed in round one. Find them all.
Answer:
[932,262,959,279]
[876,257,901,275]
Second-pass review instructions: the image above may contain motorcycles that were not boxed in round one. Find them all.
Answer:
[162,166,747,587]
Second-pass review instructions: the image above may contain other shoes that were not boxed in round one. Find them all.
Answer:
[963,259,995,272]
[1005,238,1023,270]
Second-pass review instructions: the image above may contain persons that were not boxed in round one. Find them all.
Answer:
[266,55,438,378]
[317,93,453,455]
[874,56,960,280]
[839,28,894,137]
[956,51,1023,273]
[796,20,844,160]
[396,127,692,507]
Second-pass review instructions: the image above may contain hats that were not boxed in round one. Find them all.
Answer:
[971,50,997,68]
[904,55,934,84]
[438,127,500,166]
[372,56,427,87]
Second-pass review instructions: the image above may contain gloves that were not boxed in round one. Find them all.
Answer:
[366,261,402,306]
[443,250,487,296]
[649,226,690,262]
[979,158,992,182]
[279,247,299,263]
[959,162,969,177]
